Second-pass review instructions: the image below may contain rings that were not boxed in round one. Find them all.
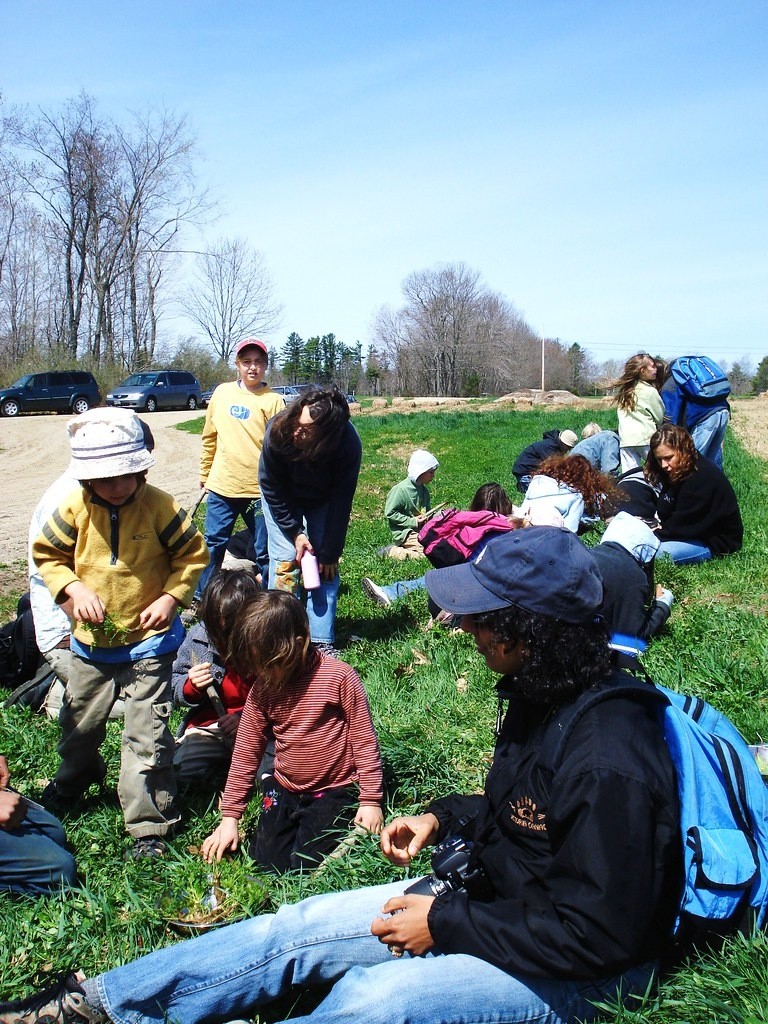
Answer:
[392,945,403,957]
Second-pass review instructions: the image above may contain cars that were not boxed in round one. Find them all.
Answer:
[197,384,221,409]
[291,385,324,399]
[269,385,301,408]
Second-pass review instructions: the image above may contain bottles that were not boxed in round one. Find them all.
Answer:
[300,550,321,590]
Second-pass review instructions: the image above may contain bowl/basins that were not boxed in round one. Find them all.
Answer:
[154,873,267,935]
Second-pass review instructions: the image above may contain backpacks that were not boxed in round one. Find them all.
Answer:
[669,353,731,404]
[418,506,516,568]
[536,649,768,965]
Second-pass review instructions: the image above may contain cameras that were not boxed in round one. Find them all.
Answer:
[389,836,494,916]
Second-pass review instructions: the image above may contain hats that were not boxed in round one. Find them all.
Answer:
[559,430,579,448]
[426,525,604,625]
[236,339,267,357]
[63,406,155,480]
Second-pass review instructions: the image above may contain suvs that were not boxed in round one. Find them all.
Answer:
[0,370,103,417]
[106,369,203,413]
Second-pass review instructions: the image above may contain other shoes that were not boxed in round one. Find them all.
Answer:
[45,758,108,804]
[125,835,166,864]
[7,663,55,711]
[362,577,389,607]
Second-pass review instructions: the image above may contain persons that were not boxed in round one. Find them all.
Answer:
[169,569,276,796]
[363,454,604,632]
[0,525,685,1024]
[586,511,675,656]
[202,589,384,874]
[258,386,362,661]
[179,338,286,628]
[515,355,744,563]
[27,406,208,861]
[0,756,74,900]
[377,451,438,558]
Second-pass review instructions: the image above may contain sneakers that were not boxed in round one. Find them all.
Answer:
[0,965,113,1024]
